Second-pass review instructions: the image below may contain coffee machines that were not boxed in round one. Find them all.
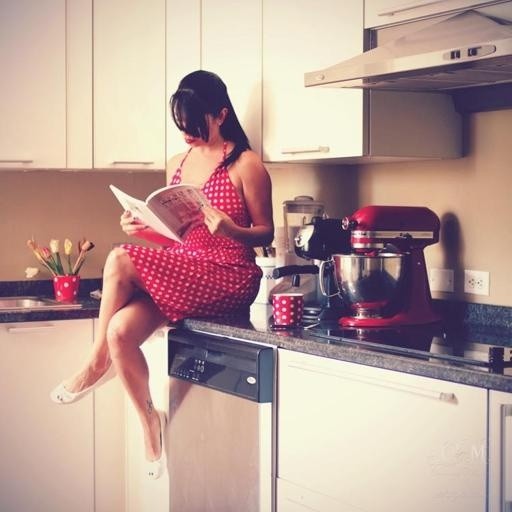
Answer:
[274,217,350,334]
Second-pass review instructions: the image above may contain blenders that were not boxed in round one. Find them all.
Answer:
[319,206,441,328]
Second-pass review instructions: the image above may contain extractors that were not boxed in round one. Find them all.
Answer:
[304,11,512,94]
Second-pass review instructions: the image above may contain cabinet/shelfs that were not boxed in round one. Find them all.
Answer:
[0,320,94,512]
[275,346,488,512]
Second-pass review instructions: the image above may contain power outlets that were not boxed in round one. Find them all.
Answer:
[464,270,489,296]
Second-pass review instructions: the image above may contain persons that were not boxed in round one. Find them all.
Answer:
[49,70,274,479]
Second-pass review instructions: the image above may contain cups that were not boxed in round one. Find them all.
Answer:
[273,293,304,329]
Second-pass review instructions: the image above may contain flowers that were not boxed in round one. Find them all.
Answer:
[27,237,94,275]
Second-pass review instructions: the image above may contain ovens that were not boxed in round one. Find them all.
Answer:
[159,330,282,512]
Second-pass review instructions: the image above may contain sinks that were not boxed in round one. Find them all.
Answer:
[0,296,85,312]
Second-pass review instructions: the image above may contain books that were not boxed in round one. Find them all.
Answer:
[109,184,209,245]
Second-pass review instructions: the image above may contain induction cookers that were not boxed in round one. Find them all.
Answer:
[318,317,511,374]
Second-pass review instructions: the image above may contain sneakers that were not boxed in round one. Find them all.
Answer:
[144,411,167,481]
[50,361,116,405]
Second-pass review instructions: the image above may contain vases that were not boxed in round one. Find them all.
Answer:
[51,275,80,303]
